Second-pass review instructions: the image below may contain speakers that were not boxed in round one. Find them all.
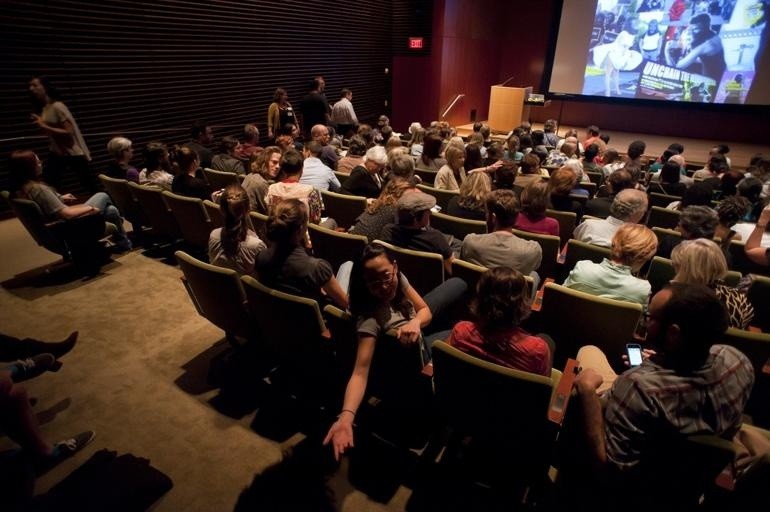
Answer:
[401,0,433,57]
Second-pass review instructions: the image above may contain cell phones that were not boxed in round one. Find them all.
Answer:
[430,206,443,213]
[626,343,643,369]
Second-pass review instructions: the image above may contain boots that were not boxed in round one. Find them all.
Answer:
[13,330,81,373]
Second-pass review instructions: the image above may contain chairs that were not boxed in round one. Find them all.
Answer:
[11,198,118,281]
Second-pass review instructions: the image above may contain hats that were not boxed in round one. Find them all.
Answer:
[394,189,440,212]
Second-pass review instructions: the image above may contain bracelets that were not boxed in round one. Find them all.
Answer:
[88,203,95,216]
[341,406,355,419]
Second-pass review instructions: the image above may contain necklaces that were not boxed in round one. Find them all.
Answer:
[280,103,287,112]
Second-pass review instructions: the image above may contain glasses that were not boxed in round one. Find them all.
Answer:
[365,262,399,288]
[642,307,668,325]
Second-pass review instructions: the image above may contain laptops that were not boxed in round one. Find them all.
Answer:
[529,93,545,100]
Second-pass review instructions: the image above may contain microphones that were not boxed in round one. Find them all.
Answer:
[502,76,515,86]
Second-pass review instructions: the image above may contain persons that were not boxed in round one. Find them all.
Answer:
[513,176,562,234]
[351,175,419,242]
[171,144,210,203]
[240,144,282,210]
[12,148,132,253]
[281,121,303,150]
[211,133,243,177]
[207,184,267,278]
[308,125,339,163]
[577,122,720,219]
[185,120,212,166]
[410,117,516,187]
[358,111,408,146]
[746,201,770,269]
[324,127,342,156]
[595,1,744,103]
[276,132,296,156]
[516,115,577,193]
[558,221,660,327]
[571,188,652,250]
[343,144,392,191]
[573,277,757,473]
[334,87,358,138]
[459,189,544,298]
[262,148,337,229]
[0,369,94,469]
[386,150,403,180]
[322,240,468,465]
[239,123,261,153]
[265,86,300,139]
[720,136,769,241]
[301,136,337,191]
[442,169,493,218]
[3,332,80,383]
[650,235,758,331]
[377,189,463,284]
[28,71,97,194]
[105,134,136,183]
[374,153,422,201]
[449,263,556,378]
[254,197,353,318]
[139,141,174,191]
[659,204,733,271]
[298,74,332,143]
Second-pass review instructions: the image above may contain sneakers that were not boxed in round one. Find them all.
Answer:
[51,430,98,465]
[8,353,56,383]
